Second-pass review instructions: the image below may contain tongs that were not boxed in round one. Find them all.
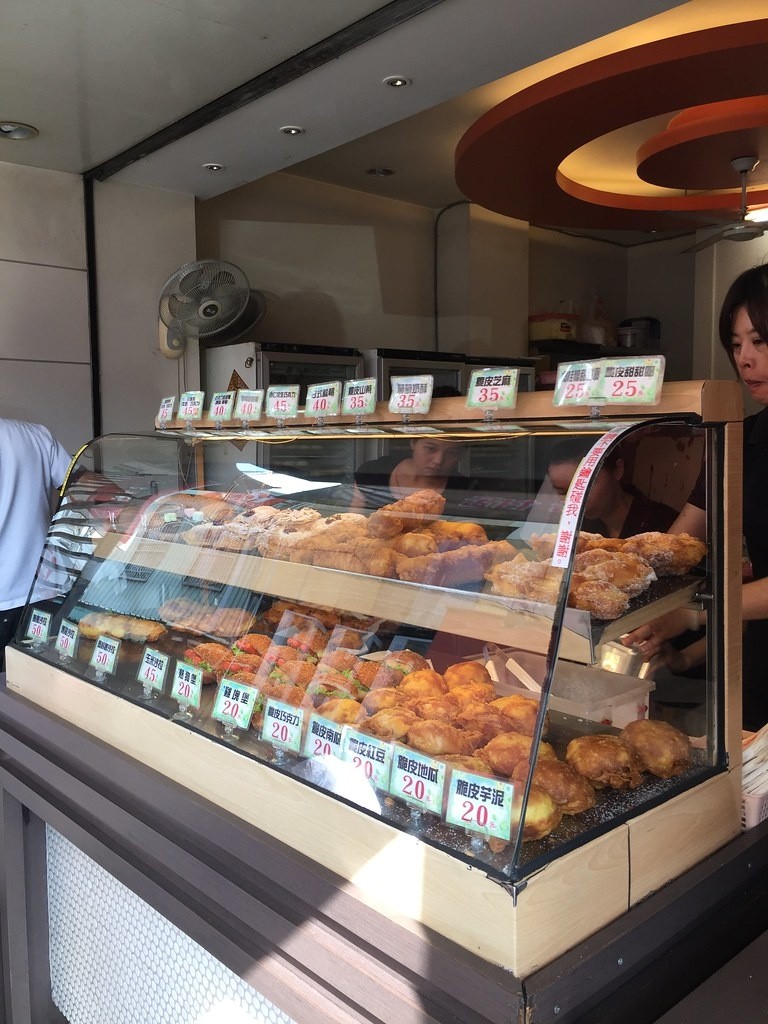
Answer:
[481,642,543,695]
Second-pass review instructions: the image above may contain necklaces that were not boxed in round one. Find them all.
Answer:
[395,459,447,498]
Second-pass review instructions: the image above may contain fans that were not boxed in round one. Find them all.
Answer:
[669,155,768,255]
[156,258,253,359]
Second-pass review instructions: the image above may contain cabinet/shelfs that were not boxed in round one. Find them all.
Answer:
[0,377,767,1024]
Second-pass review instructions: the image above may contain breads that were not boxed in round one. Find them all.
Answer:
[64,486,710,850]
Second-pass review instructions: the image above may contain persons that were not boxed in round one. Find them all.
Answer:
[545,434,747,678]
[341,436,478,517]
[0,418,132,673]
[618,262,768,716]
